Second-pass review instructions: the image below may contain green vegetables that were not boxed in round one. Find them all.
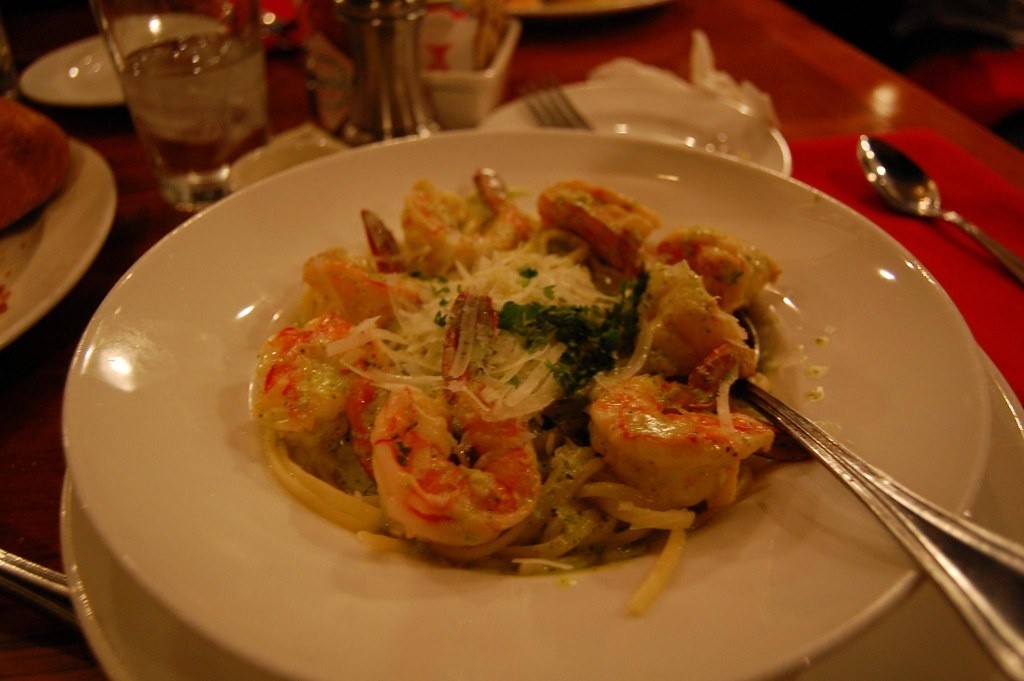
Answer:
[404,254,743,464]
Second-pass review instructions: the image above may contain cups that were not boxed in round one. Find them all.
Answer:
[92,0,268,212]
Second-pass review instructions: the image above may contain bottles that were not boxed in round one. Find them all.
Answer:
[0,19,18,100]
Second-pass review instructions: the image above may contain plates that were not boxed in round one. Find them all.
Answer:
[61,325,1024,681]
[18,13,217,106]
[63,128,990,681]
[0,138,117,352]
[475,77,793,178]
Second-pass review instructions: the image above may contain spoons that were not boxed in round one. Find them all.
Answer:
[859,133,1024,288]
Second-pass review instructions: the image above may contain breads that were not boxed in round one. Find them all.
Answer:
[0,96,69,233]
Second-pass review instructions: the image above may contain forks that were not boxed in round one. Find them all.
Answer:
[519,82,598,132]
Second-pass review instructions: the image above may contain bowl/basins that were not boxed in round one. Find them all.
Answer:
[418,10,522,124]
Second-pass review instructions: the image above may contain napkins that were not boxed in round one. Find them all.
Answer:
[789,128,1024,412]
